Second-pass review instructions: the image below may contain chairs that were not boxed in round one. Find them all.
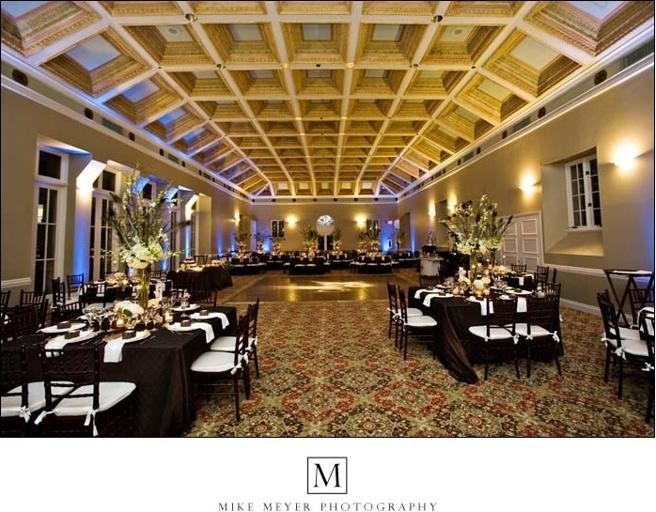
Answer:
[387,263,564,380]
[597,287,655,424]
[194,251,419,274]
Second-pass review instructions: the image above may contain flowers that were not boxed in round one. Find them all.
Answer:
[90,161,193,310]
[232,223,409,264]
[438,193,513,283]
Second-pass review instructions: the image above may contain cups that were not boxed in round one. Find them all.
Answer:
[93,269,168,286]
[38,287,218,344]
[434,265,554,300]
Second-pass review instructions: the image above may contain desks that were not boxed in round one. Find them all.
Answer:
[603,268,654,328]
